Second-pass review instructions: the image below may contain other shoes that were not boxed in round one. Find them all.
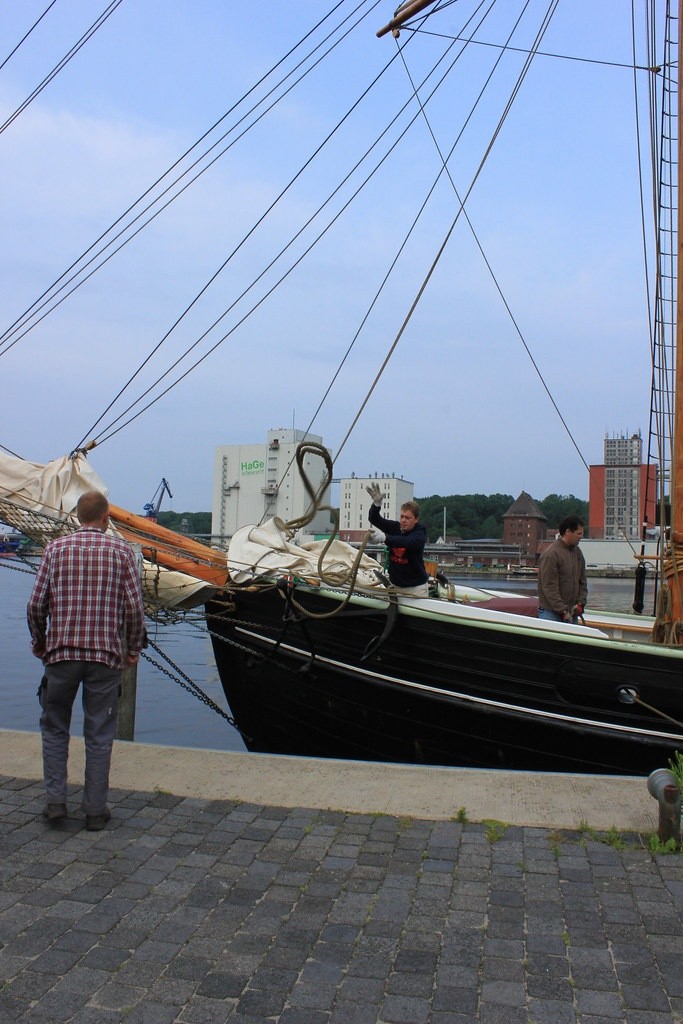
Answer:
[85,805,110,831]
[43,802,66,818]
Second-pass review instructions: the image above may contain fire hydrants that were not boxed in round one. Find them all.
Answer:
[648,769,683,851]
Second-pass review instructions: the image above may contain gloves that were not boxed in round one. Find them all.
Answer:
[366,481,386,507]
[571,603,584,617]
[563,610,573,623]
[368,528,387,544]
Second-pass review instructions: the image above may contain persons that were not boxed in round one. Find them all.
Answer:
[26,491,144,833]
[364,482,430,598]
[536,517,588,622]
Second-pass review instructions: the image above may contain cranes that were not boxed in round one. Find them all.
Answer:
[143,477,173,518]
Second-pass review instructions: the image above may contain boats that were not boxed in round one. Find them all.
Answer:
[513,566,540,577]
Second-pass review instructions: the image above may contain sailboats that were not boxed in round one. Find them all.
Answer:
[0,0,683,778]
